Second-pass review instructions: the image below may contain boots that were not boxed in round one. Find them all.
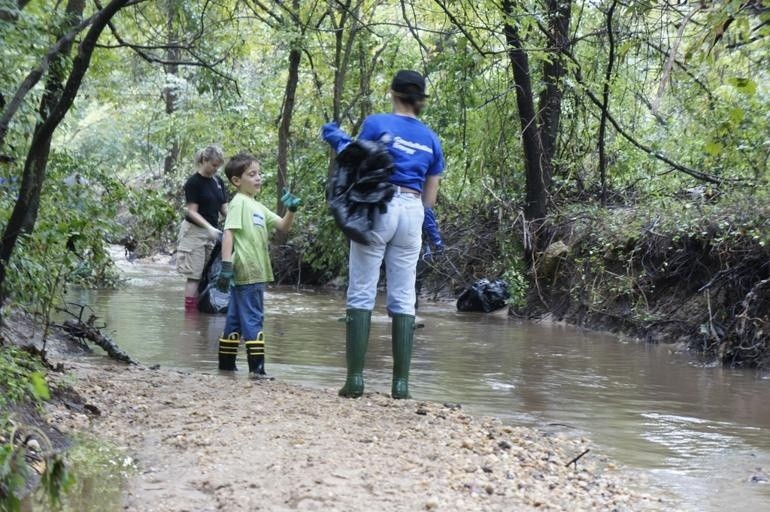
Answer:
[246,330,276,380]
[392,312,414,399]
[337,307,371,396]
[185,296,200,315]
[219,329,240,372]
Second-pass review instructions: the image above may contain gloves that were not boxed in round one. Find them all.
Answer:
[427,226,444,254]
[217,261,234,293]
[323,122,351,150]
[280,187,301,212]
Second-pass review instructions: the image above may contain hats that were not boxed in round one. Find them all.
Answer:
[392,71,432,99]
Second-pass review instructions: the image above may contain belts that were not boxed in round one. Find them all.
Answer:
[395,187,420,194]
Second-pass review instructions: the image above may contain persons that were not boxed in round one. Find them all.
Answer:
[176,144,229,314]
[214,153,302,381]
[321,69,447,400]
